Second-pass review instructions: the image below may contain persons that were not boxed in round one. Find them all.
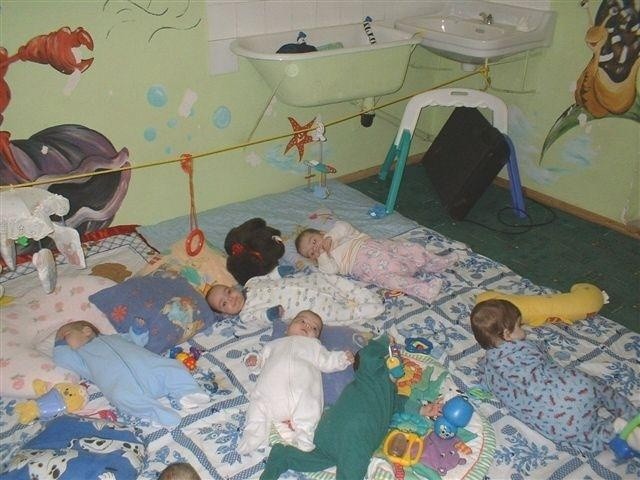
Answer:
[258,329,445,479]
[233,309,356,455]
[50,315,212,432]
[157,462,201,480]
[469,298,640,454]
[203,264,387,329]
[294,220,459,304]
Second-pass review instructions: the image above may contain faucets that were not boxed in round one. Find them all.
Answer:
[479,12,493,24]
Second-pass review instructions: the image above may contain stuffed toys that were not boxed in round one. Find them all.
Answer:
[13,378,85,426]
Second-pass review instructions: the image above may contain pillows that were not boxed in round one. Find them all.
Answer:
[89,264,218,355]
[132,234,240,298]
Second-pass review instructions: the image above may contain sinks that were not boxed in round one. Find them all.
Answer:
[393,0,559,66]
[229,21,425,108]
